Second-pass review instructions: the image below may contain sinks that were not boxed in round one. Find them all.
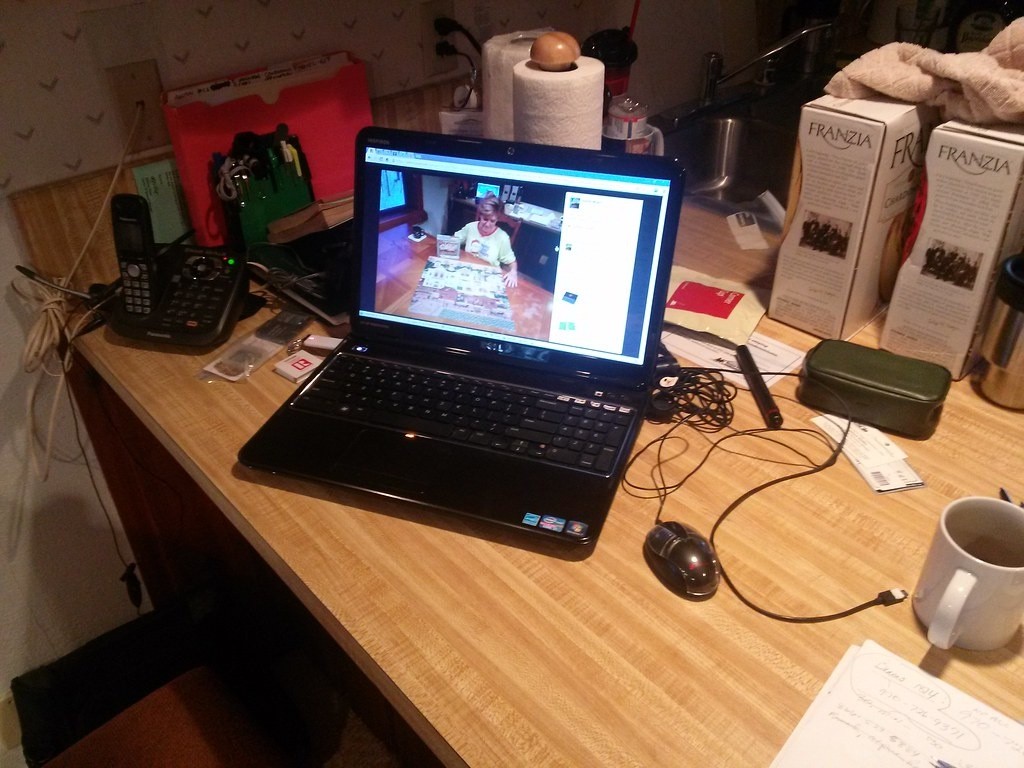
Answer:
[648,96,838,235]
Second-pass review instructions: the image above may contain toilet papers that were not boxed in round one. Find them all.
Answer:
[480,30,563,144]
[511,56,607,154]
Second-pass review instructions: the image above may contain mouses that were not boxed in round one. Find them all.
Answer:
[643,520,721,599]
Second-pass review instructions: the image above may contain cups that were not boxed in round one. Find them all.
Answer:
[910,496,1024,650]
[601,123,665,157]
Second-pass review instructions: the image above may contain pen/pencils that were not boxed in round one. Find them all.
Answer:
[735,344,785,428]
[211,122,304,209]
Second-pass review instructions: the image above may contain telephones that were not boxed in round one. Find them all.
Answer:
[108,193,251,348]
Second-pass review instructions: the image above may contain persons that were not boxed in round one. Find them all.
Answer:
[450,195,518,289]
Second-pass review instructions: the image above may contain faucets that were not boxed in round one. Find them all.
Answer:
[696,11,841,113]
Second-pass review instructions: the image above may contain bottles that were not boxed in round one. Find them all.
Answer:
[606,94,648,139]
[972,248,1024,411]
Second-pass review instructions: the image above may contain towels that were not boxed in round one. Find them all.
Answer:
[823,13,1024,132]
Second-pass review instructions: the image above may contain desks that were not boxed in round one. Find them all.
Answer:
[63,174,1024,768]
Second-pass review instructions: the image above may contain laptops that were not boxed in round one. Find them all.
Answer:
[236,126,687,546]
